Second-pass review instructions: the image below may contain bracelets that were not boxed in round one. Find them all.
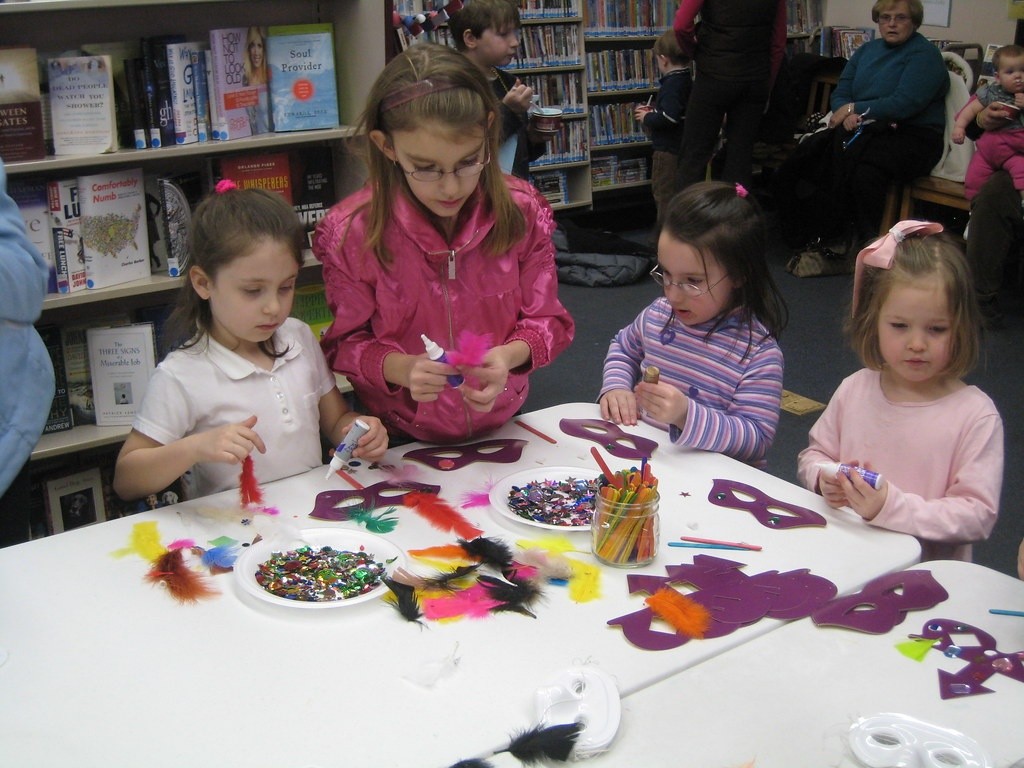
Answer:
[975,110,983,128]
[848,103,852,116]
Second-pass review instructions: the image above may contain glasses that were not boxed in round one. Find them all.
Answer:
[877,14,913,24]
[650,263,731,297]
[387,133,491,182]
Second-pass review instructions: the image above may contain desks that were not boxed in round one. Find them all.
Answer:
[517,555,1024,768]
[0,402,921,768]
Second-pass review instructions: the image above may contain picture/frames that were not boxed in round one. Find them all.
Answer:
[30,461,119,537]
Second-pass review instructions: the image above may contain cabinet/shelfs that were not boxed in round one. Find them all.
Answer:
[0,0,396,461]
[589,0,826,191]
[395,0,593,228]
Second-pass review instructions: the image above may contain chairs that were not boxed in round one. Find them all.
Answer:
[712,41,982,267]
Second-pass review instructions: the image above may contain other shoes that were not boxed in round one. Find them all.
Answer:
[972,291,1005,330]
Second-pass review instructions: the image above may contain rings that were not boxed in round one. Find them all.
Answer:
[830,127,833,129]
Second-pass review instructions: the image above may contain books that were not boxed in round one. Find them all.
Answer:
[786,0,965,61]
[0,26,338,544]
[391,0,698,209]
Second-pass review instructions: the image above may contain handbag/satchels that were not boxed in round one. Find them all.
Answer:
[786,215,857,279]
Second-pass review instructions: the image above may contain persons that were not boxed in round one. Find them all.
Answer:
[952,46,1024,331]
[597,182,785,463]
[0,157,56,498]
[312,43,575,449]
[796,219,1003,564]
[113,179,389,501]
[634,30,693,253]
[674,0,787,194]
[242,25,270,136]
[768,0,951,261]
[447,0,565,183]
